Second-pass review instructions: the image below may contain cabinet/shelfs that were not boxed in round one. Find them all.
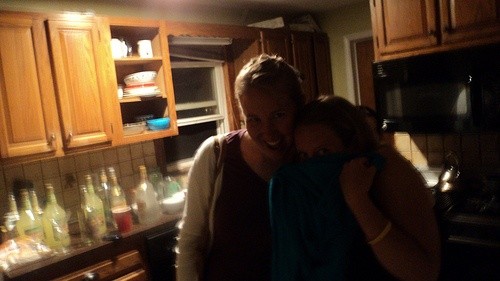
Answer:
[0,9,118,167]
[102,16,179,145]
[230,27,334,124]
[8,234,152,281]
[368,0,500,63]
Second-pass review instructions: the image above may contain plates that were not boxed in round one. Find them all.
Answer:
[124,91,160,97]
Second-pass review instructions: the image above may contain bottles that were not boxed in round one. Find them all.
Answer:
[135,165,160,225]
[77,185,101,244]
[17,189,45,243]
[108,169,127,208]
[149,167,163,196]
[1,192,20,243]
[84,174,108,235]
[95,169,112,225]
[28,188,47,246]
[112,38,128,58]
[43,183,70,249]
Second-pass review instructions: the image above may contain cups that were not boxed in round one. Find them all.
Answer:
[111,207,132,234]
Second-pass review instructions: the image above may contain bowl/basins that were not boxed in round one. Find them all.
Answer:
[124,70,156,86]
[123,122,146,135]
[147,118,170,131]
[162,196,185,215]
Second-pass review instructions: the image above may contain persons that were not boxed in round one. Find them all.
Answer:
[175,53,306,281]
[293,94,441,281]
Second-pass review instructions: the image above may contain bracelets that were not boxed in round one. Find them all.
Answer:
[368,221,393,246]
